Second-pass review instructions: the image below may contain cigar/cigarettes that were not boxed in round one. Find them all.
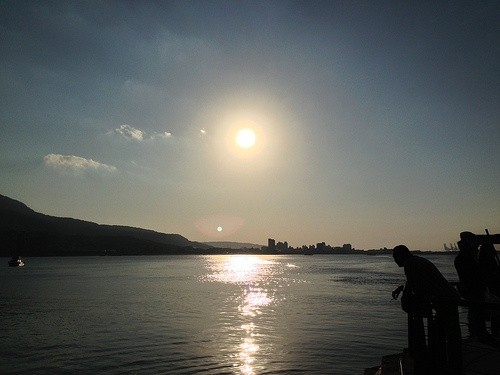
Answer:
[391,297,397,300]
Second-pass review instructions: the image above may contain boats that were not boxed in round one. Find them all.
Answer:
[7,256,25,269]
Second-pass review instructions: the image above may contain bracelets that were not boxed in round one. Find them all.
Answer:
[397,285,403,291]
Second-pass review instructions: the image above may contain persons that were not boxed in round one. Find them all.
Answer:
[453,231,491,342]
[476,238,500,342]
[391,245,464,375]
